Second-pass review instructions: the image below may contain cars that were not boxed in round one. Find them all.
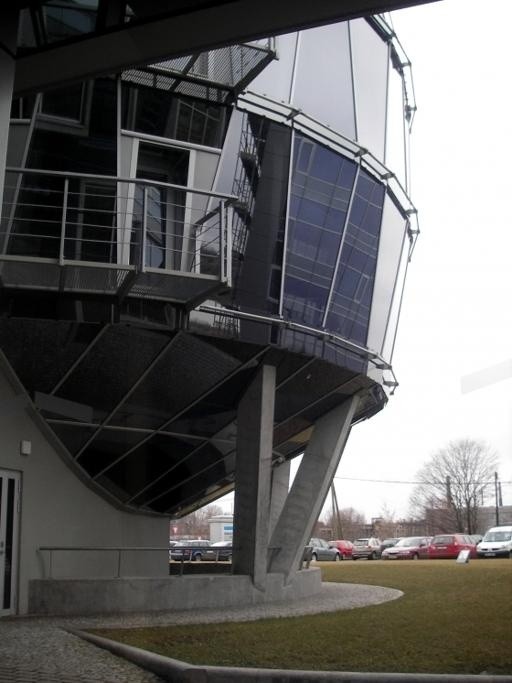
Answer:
[305,533,474,561]
[170,540,233,561]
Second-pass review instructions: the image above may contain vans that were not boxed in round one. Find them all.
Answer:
[475,526,512,557]
